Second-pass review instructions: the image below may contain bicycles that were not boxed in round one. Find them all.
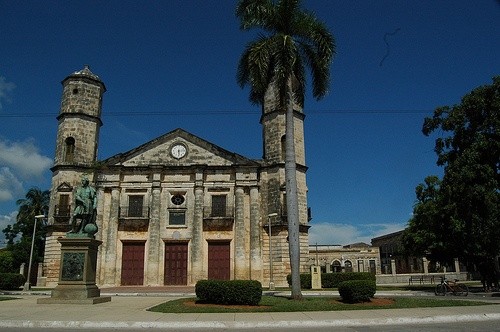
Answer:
[435,276,468,297]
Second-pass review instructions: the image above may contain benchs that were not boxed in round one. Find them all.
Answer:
[409,273,448,286]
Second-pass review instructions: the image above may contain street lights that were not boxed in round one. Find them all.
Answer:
[268,213,278,291]
[23,214,46,291]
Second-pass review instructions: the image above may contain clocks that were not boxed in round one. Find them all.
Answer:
[169,140,189,161]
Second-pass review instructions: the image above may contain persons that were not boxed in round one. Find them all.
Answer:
[70,176,98,238]
